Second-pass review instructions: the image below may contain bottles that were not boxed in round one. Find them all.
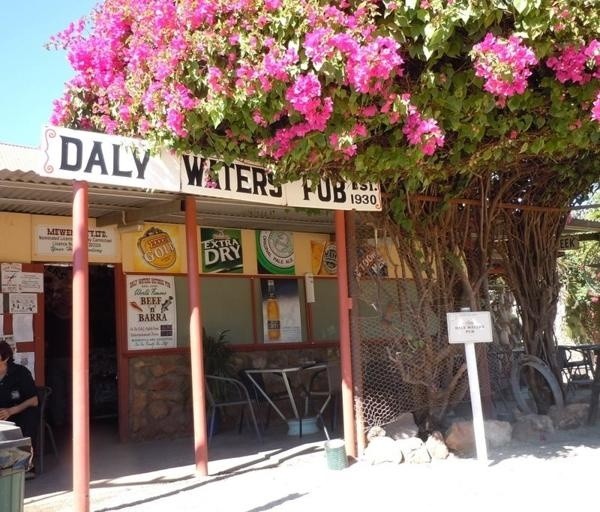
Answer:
[316,233,338,276]
[266,279,281,341]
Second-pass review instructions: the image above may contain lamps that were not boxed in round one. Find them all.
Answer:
[116,221,147,235]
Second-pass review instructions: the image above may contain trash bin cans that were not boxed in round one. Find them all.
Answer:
[0,420,34,512]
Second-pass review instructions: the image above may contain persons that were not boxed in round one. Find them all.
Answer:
[0,339,40,445]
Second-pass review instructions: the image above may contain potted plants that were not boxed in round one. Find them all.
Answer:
[201,325,239,437]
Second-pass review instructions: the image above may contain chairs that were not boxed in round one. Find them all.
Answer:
[201,357,342,449]
[17,386,58,476]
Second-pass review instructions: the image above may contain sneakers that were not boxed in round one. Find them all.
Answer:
[25,462,36,480]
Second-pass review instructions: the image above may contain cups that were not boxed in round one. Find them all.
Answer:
[311,239,327,275]
